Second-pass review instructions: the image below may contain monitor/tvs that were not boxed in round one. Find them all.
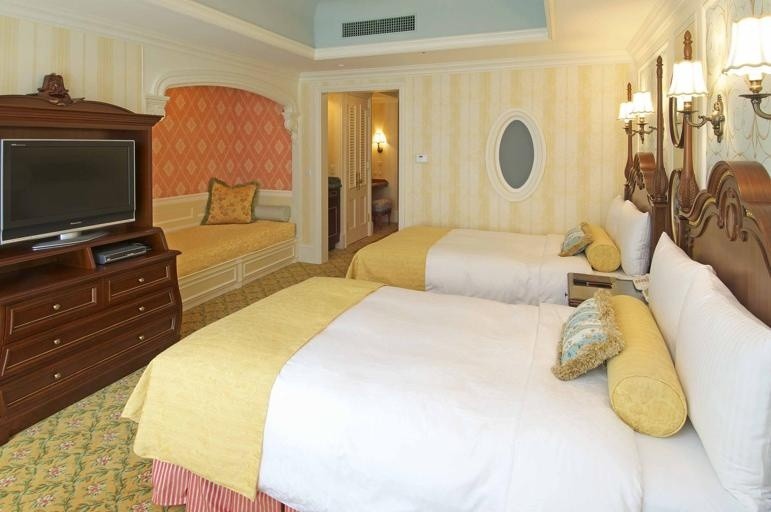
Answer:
[0,139,136,250]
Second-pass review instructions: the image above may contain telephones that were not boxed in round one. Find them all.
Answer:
[633,274,650,290]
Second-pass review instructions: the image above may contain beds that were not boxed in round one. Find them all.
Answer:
[343,53,670,306]
[161,215,299,313]
[124,31,771,512]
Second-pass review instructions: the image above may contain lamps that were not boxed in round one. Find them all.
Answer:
[374,130,386,153]
[628,91,657,143]
[617,101,640,137]
[668,58,726,146]
[720,15,771,120]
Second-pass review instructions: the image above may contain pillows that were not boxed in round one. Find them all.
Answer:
[618,199,652,279]
[582,222,618,271]
[552,291,625,381]
[559,227,592,257]
[251,203,292,224]
[673,263,771,512]
[604,194,625,249]
[200,176,259,228]
[646,232,717,366]
[605,296,687,439]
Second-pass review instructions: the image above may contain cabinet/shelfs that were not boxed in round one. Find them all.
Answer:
[0,72,183,446]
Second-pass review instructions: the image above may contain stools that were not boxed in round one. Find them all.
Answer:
[372,198,391,225]
